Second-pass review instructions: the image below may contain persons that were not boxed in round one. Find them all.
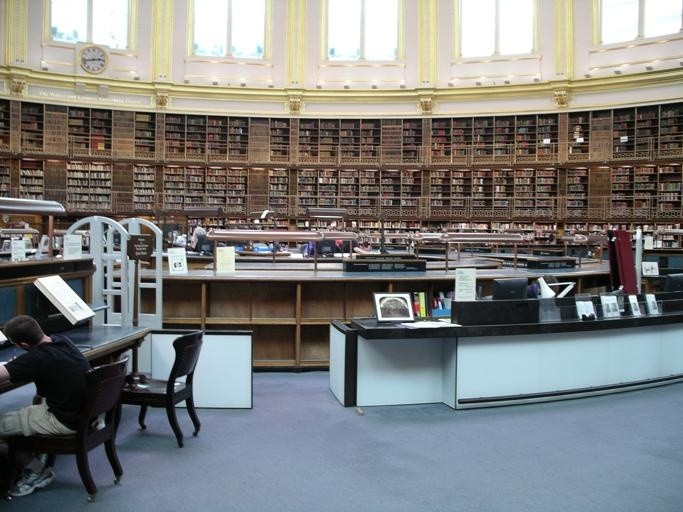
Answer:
[190,224,212,253]
[0,315,99,496]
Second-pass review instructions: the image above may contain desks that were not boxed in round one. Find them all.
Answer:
[2,327,155,411]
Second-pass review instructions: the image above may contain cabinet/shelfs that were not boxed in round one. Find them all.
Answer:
[0,264,97,340]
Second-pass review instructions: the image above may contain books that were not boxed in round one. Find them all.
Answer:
[1,97,683,154]
[0,158,681,216]
[188,219,682,250]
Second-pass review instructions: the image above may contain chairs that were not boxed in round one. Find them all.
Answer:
[112,330,204,448]
[6,355,129,503]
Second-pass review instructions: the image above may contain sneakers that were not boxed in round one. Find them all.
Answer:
[8,467,56,498]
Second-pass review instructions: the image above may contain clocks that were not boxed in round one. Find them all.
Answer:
[78,43,111,76]
